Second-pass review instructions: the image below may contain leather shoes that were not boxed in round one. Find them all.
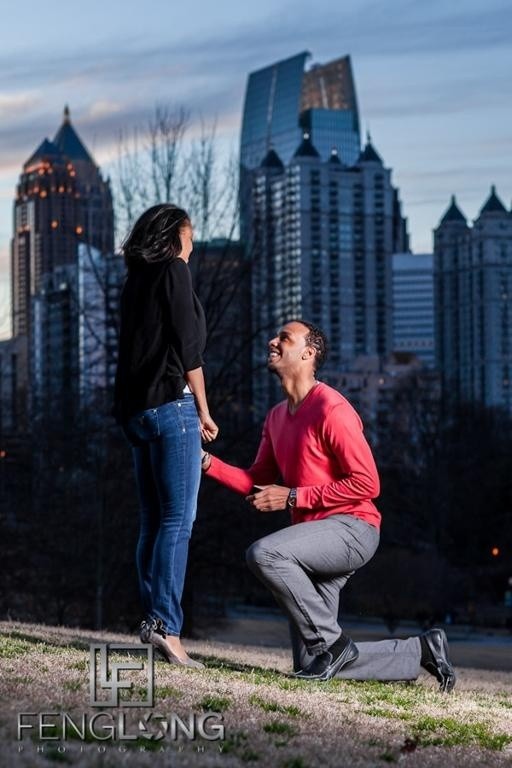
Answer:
[286,639,360,683]
[423,627,455,693]
[140,624,207,673]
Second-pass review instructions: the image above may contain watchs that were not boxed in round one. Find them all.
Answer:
[286,486,297,509]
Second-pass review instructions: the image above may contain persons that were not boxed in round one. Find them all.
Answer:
[200,317,457,694]
[111,203,220,671]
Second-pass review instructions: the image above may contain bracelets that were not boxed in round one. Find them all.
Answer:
[201,451,210,464]
